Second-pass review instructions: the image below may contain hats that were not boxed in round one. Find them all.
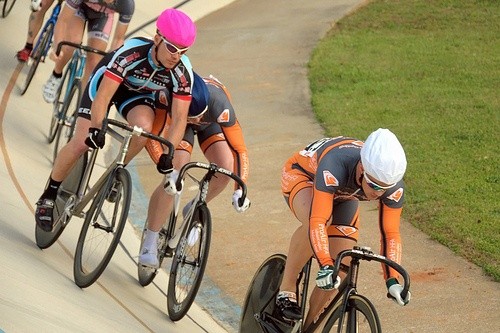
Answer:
[186,72,210,119]
[156,8,196,48]
[360,128,407,185]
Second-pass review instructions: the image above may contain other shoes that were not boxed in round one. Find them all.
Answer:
[34,193,56,232]
[182,199,198,247]
[16,47,31,64]
[139,228,160,266]
[42,74,62,103]
[106,183,122,203]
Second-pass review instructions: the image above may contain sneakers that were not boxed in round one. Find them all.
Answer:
[276,291,303,320]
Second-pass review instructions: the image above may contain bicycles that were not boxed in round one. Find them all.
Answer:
[46,40,112,161]
[241,248,413,333]
[34,118,177,289]
[21,0,70,95]
[2,0,16,18]
[137,161,248,323]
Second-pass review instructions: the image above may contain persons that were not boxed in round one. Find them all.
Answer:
[17,0,252,270]
[279,130,411,333]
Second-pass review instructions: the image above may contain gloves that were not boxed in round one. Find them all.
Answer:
[84,127,106,150]
[315,265,341,291]
[386,277,411,307]
[157,153,174,174]
[232,189,251,213]
[162,169,185,196]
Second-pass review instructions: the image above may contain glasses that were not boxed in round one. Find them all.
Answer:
[161,38,189,57]
[363,172,396,192]
